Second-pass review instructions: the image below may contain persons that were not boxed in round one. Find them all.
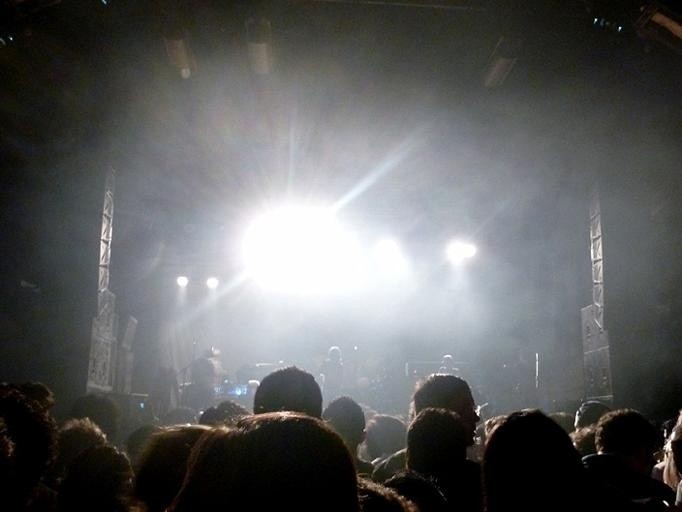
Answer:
[1,347,681,512]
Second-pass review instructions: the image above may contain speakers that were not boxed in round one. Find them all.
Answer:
[72,151,120,315]
[586,158,631,330]
